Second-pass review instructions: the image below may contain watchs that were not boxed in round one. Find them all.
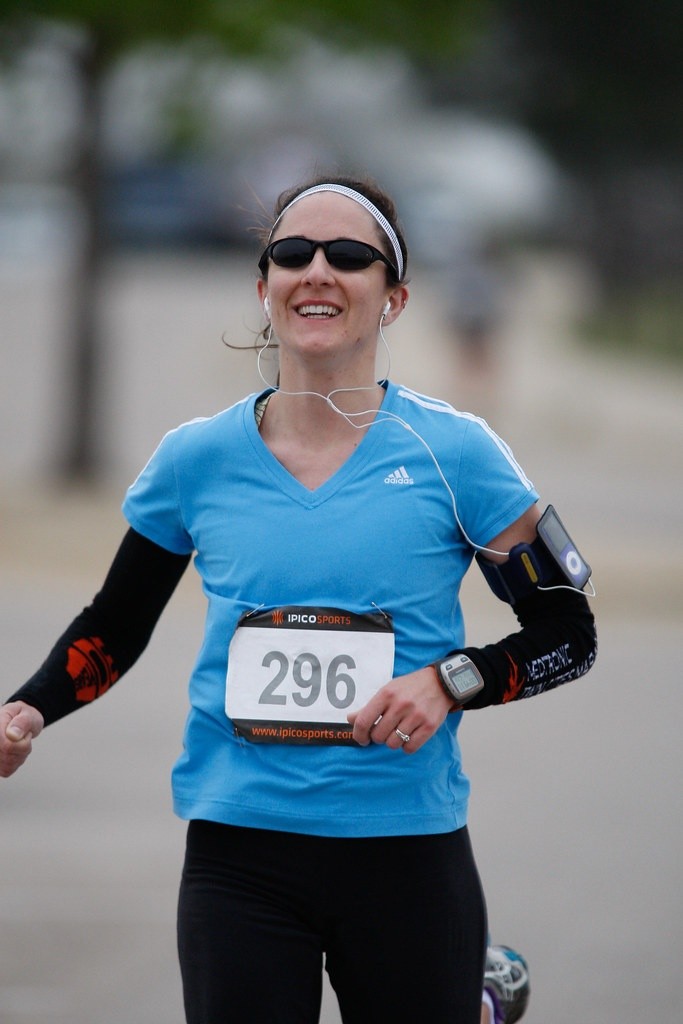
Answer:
[428,654,484,713]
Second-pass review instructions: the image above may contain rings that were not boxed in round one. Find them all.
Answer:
[395,729,409,742]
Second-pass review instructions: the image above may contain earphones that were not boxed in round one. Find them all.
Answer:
[264,296,271,319]
[382,301,390,319]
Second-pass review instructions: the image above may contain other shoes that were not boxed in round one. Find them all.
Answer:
[482,944,529,1024]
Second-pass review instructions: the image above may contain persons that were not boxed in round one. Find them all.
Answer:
[0,178,598,1024]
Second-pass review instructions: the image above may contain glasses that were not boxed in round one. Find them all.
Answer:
[265,238,400,277]
